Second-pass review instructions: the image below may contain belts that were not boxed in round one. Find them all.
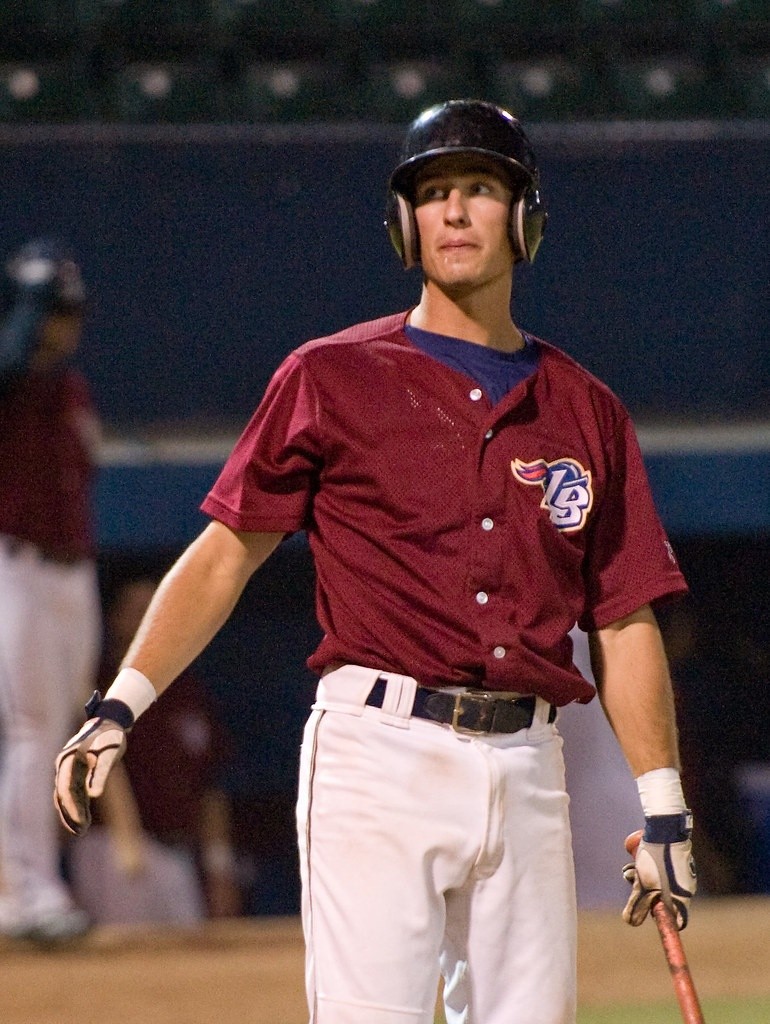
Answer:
[366,680,558,737]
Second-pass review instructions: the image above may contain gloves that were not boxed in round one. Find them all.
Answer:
[53,689,136,838]
[619,811,700,933]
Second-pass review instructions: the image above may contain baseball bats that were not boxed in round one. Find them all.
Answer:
[621,823,704,1024]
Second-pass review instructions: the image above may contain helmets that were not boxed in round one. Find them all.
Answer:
[383,99,545,272]
[4,246,87,306]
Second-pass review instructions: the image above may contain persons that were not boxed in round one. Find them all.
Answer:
[0,238,239,943]
[52,100,699,1024]
